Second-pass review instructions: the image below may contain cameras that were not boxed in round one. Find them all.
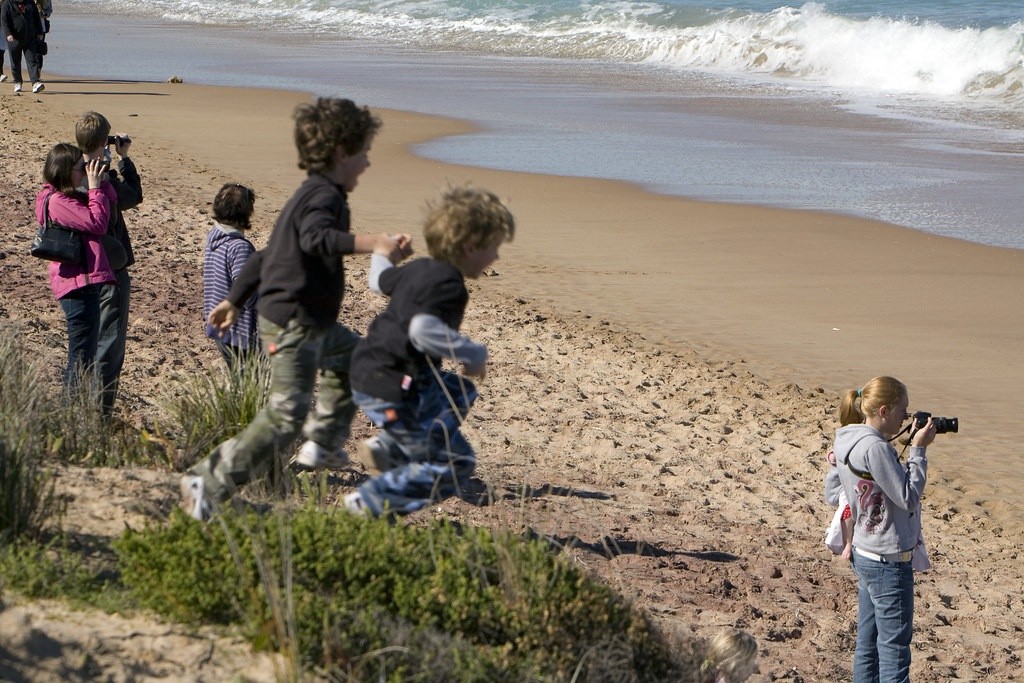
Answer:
[913,411,958,434]
[108,136,124,147]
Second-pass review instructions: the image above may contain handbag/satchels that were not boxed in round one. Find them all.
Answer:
[36,39,48,55]
[31,192,85,264]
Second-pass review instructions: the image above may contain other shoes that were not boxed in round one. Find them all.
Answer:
[0,75,8,82]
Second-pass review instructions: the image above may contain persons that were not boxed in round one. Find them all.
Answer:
[204,184,262,372]
[823,375,937,683]
[343,186,516,527]
[34,110,143,416]
[0,0,53,93]
[184,98,415,523]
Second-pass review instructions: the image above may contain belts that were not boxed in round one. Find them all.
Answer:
[853,544,913,562]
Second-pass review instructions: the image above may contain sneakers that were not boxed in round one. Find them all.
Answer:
[179,473,213,522]
[32,82,45,93]
[14,82,22,92]
[361,436,396,479]
[344,490,372,519]
[297,439,350,468]
